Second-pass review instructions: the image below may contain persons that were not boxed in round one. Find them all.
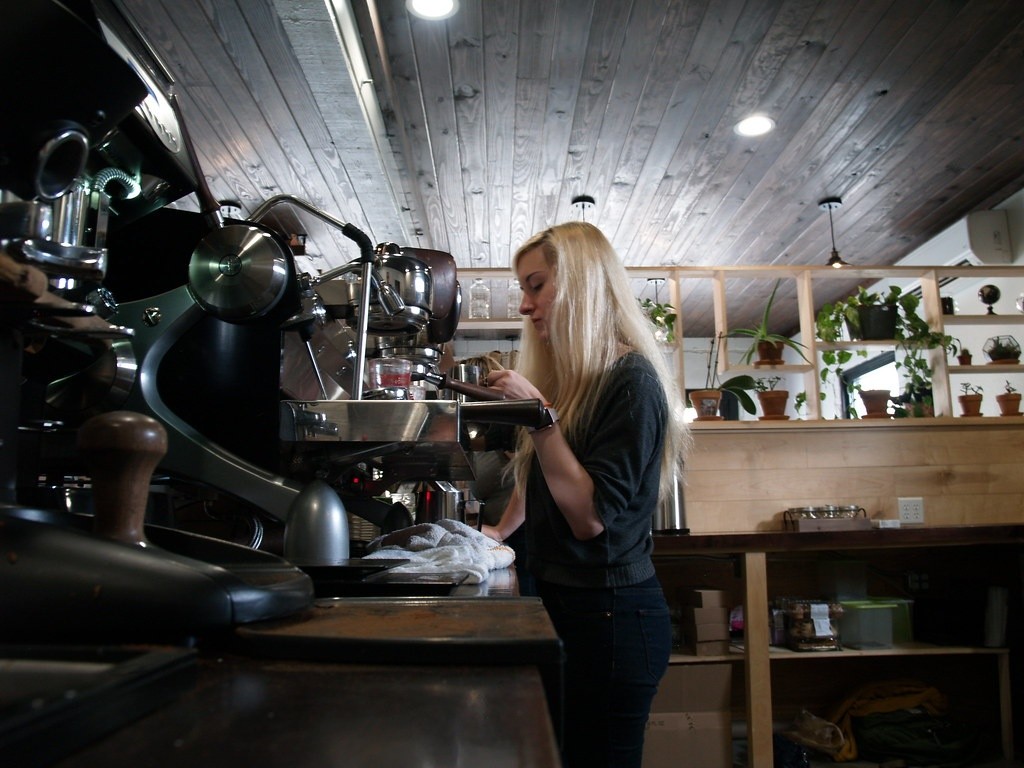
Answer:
[470,222,697,768]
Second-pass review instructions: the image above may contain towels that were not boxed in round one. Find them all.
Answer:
[361,518,516,585]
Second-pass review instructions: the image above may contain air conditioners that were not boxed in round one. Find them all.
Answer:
[890,208,1013,310]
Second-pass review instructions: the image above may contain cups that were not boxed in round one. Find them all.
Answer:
[363,358,413,400]
[446,365,484,403]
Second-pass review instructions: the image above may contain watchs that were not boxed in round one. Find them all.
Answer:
[524,408,560,434]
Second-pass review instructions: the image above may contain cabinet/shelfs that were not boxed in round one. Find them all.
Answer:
[456,267,1024,768]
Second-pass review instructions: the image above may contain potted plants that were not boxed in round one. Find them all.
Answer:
[957,349,973,365]
[688,331,760,422]
[794,285,960,419]
[755,375,791,420]
[996,380,1024,415]
[856,386,893,419]
[957,383,984,416]
[719,278,813,367]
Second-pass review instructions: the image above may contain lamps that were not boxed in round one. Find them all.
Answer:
[818,197,851,267]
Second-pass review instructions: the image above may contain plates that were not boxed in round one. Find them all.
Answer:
[789,505,858,518]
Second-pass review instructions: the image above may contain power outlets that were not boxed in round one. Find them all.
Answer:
[899,498,924,523]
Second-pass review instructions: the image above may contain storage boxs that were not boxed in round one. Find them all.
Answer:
[641,663,733,768]
[836,603,898,650]
[686,588,730,657]
